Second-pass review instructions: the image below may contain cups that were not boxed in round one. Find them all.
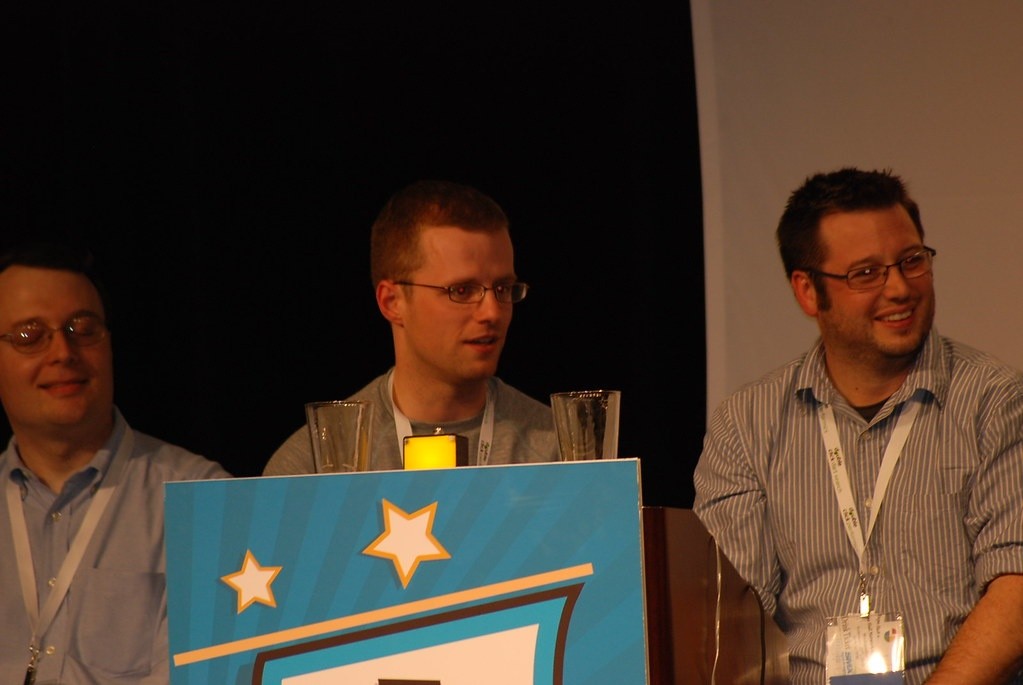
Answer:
[304,401,371,476]
[551,390,618,463]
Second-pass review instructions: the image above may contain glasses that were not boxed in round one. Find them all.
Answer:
[804,246,936,290]
[0,315,109,354]
[393,280,529,304]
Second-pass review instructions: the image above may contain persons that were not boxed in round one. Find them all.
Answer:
[262,179,594,476]
[0,245,231,685]
[693,166,1023,685]
